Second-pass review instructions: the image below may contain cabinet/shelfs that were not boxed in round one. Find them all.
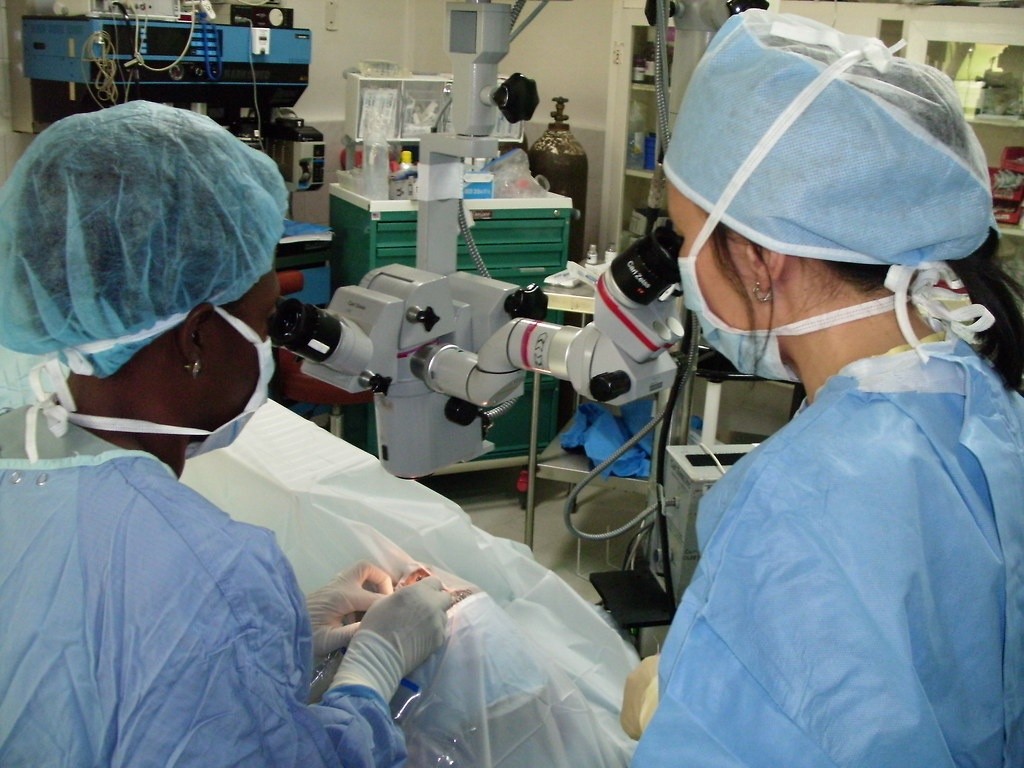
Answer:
[327,182,572,497]
[520,261,707,650]
[591,0,1023,400]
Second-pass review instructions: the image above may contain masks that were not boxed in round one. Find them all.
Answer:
[174,307,277,459]
[677,210,836,382]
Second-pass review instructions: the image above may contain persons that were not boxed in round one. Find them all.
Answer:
[619,7,1024,767]
[0,100,451,768]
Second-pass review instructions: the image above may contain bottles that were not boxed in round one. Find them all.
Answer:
[632,54,645,84]
[644,52,654,85]
[604,243,616,263]
[586,244,598,265]
[644,131,656,170]
[399,151,413,169]
